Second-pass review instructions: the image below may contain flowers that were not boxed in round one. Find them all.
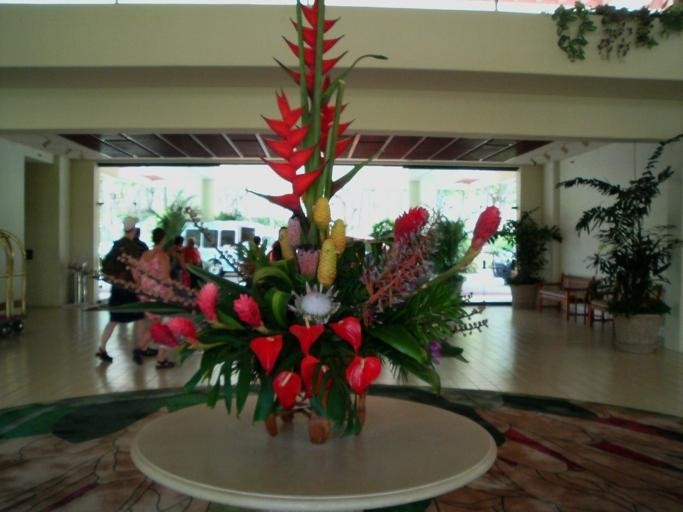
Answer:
[69,0,502,439]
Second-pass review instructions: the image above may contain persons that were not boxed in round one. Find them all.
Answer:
[93,212,288,368]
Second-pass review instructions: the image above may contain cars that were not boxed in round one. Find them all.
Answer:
[493,248,517,280]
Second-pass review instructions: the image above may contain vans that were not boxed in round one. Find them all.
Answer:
[178,220,278,277]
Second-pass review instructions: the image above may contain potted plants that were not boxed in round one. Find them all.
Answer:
[553,131,683,354]
[480,203,563,309]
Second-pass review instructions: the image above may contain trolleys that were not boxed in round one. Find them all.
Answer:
[0,231,28,336]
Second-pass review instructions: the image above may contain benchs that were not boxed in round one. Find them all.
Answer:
[534,274,666,337]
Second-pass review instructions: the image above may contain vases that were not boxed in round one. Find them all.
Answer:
[263,360,370,444]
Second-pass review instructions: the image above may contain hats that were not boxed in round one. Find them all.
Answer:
[123,217,138,231]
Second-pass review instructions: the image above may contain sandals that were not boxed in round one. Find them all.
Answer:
[94,347,112,362]
[133,346,172,368]
